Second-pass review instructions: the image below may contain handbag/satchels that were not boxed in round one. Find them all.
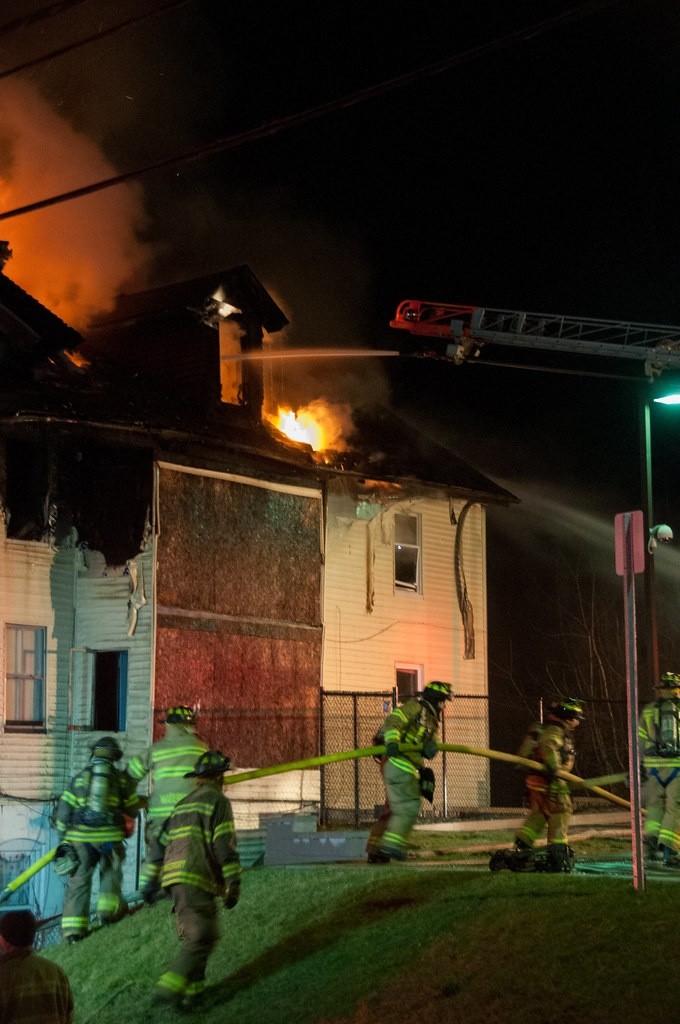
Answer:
[418,767,435,804]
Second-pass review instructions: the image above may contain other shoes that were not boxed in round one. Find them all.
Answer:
[643,838,660,861]
[67,934,83,945]
[367,846,407,865]
[100,912,125,925]
[143,892,155,906]
[662,846,680,866]
[155,993,192,1015]
[514,838,532,851]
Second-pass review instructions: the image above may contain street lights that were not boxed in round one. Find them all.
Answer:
[642,381,679,692]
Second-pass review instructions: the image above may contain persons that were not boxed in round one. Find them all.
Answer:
[152,747,243,1008]
[49,702,216,948]
[639,671,680,867]
[0,907,74,1024]
[511,695,587,860]
[366,680,455,864]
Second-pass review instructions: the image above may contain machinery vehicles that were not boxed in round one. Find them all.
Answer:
[386,291,680,388]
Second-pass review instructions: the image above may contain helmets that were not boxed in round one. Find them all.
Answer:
[183,750,231,778]
[91,736,124,760]
[423,680,454,701]
[551,698,584,719]
[652,671,680,689]
[159,704,196,725]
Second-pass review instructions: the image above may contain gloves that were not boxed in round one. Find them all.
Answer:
[423,739,437,760]
[222,881,240,910]
[385,742,399,758]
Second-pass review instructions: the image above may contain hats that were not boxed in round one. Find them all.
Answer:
[0,909,37,946]
